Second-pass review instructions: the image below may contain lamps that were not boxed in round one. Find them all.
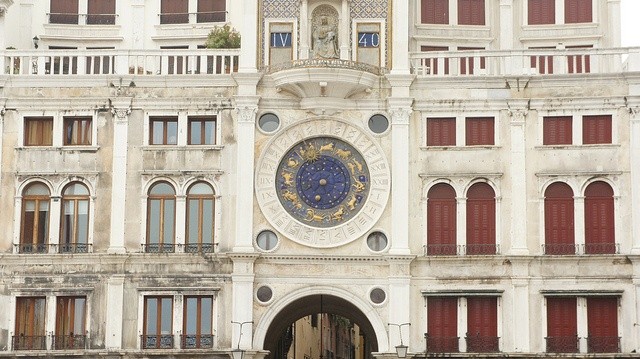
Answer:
[32,37,39,50]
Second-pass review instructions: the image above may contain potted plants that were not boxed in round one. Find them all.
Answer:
[205,21,241,74]
